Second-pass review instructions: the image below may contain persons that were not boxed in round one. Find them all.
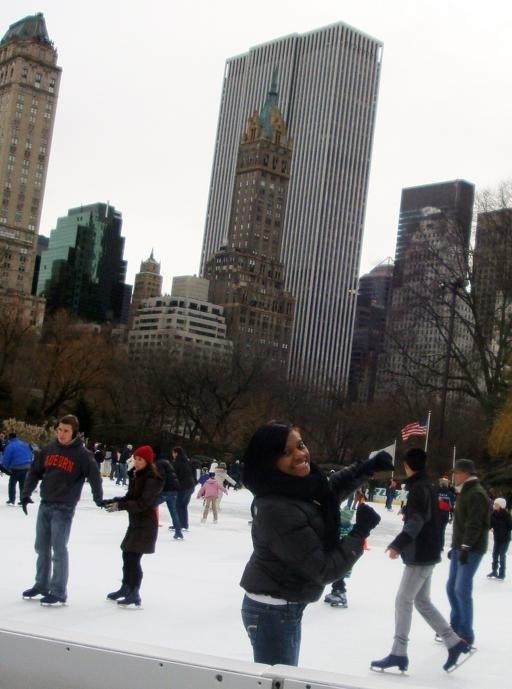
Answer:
[434,475,458,551]
[324,466,407,608]
[485,497,512,582]
[0,414,242,609]
[370,446,471,671]
[437,457,491,647]
[237,422,399,669]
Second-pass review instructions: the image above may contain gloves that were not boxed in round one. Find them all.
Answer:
[94,497,119,512]
[366,451,395,478]
[22,499,34,515]
[459,550,470,564]
[354,502,381,533]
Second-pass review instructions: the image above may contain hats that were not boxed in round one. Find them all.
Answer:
[133,445,155,465]
[450,458,477,474]
[494,498,507,509]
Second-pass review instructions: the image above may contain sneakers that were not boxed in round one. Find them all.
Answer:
[497,574,505,579]
[118,593,141,605]
[324,589,348,603]
[40,594,66,603]
[108,585,127,599]
[169,525,175,529]
[488,571,497,576]
[371,654,408,671]
[174,530,182,538]
[7,499,15,504]
[18,501,22,504]
[23,587,49,597]
[443,638,470,671]
